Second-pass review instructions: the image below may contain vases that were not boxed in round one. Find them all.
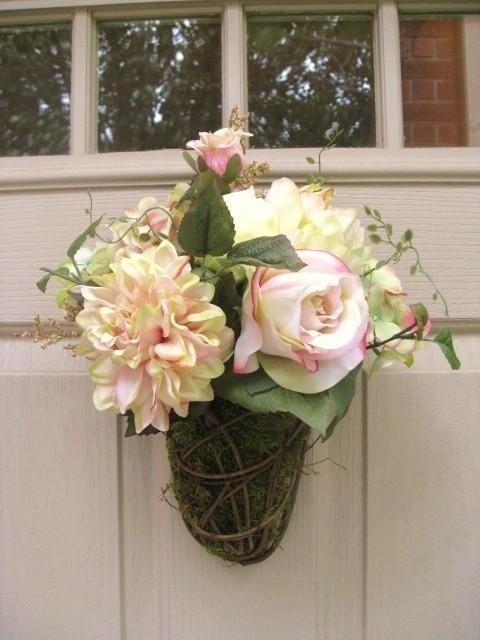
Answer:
[165,398,311,565]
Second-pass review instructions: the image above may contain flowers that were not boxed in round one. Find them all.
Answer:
[13,106,461,436]
